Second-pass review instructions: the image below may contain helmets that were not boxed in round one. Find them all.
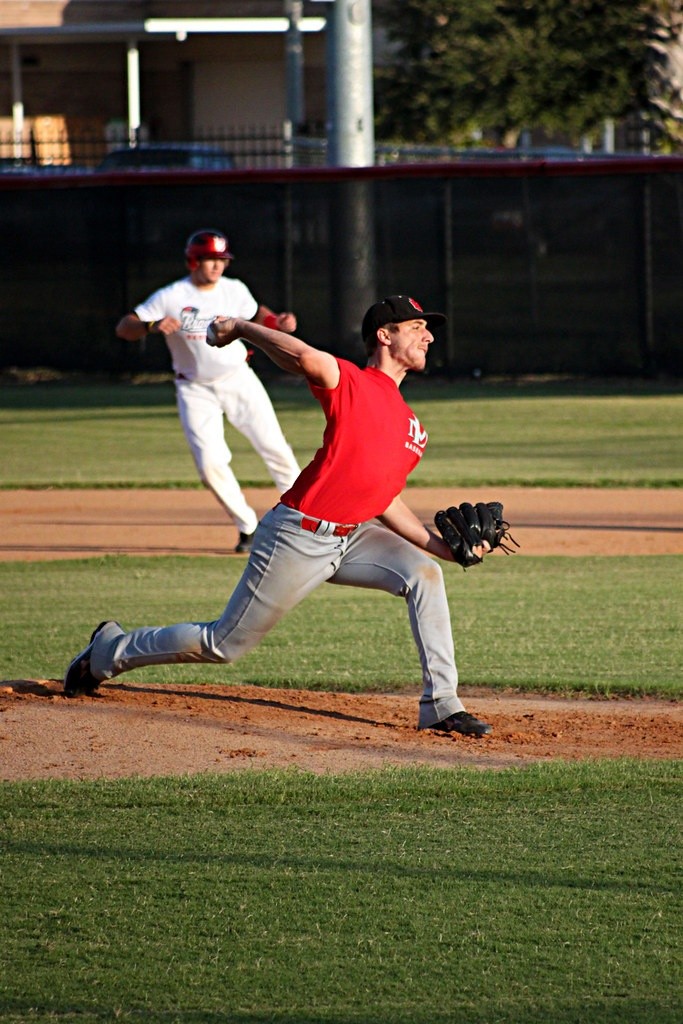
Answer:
[185,232,233,273]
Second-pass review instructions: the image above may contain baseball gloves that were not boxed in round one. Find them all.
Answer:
[433,501,506,568]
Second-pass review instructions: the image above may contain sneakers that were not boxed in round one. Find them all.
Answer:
[64,621,120,697]
[431,711,488,736]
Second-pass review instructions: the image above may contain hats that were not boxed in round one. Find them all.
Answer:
[359,294,442,341]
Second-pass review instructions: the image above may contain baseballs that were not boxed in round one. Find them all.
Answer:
[206,321,218,346]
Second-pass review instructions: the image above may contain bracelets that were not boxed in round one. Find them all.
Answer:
[145,319,158,335]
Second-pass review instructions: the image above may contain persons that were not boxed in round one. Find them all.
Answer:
[58,292,491,738]
[115,229,303,556]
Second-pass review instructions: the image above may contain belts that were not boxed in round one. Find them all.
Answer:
[273,503,357,537]
[174,373,188,380]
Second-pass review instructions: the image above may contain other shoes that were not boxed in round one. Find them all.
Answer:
[235,532,254,554]
